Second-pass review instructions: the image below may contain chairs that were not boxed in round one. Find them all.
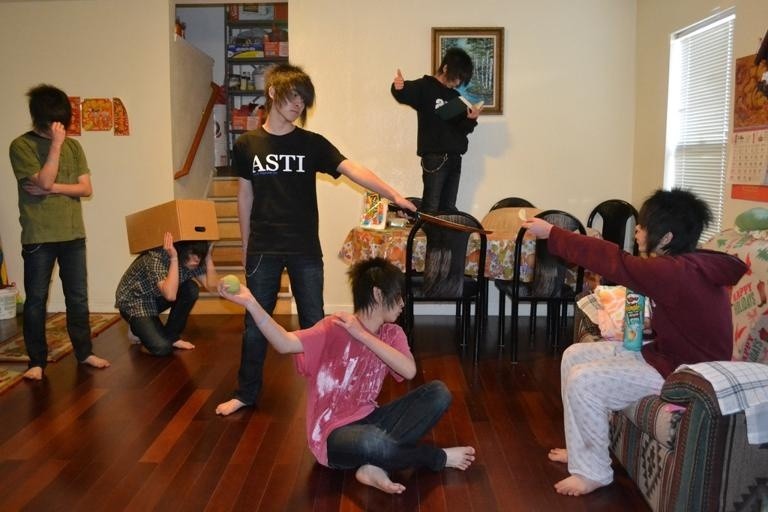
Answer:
[403,210,487,364]
[588,199,643,260]
[386,197,426,210]
[488,196,537,210]
[495,210,588,367]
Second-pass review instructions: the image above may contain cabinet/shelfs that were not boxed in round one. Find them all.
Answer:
[224,3,290,169]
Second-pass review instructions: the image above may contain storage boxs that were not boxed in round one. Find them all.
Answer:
[226,43,265,59]
[230,113,262,132]
[124,196,221,257]
[264,41,289,57]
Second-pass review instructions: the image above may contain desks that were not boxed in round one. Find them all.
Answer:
[338,224,601,301]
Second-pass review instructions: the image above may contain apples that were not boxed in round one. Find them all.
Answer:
[223,275,240,293]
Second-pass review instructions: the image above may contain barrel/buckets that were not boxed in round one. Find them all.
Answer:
[0,285,16,320]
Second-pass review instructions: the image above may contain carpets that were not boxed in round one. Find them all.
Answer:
[1,365,23,400]
[0,312,127,365]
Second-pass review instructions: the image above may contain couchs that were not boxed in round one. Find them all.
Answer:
[568,224,768,511]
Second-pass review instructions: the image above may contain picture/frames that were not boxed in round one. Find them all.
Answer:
[430,25,505,117]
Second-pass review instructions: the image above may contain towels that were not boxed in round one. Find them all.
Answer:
[743,402,768,445]
[673,360,767,416]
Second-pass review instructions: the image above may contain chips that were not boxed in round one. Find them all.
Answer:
[518,209,526,221]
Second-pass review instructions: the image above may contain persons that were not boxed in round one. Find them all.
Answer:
[114,229,220,363]
[388,45,485,231]
[211,62,420,419]
[520,186,749,498]
[8,82,111,382]
[213,257,477,494]
